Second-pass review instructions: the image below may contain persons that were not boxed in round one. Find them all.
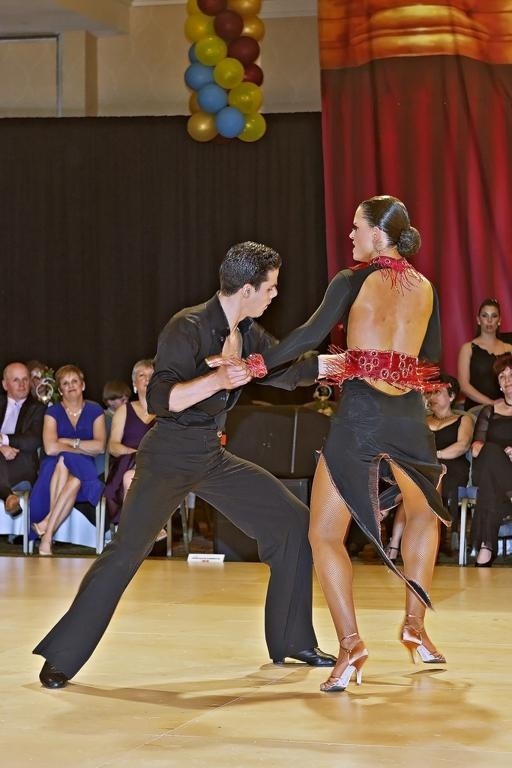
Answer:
[1,299,511,568]
[205,192,454,691]
[32,238,338,690]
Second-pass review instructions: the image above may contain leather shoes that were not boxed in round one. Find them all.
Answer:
[40,661,68,688]
[273,647,338,666]
[4,494,22,519]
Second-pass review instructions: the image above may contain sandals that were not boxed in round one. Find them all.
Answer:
[400,615,447,664]
[320,633,368,691]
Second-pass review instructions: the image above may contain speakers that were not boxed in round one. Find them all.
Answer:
[213,478,310,562]
[222,404,331,479]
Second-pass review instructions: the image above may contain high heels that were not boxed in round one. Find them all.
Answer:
[475,546,497,567]
[38,544,53,556]
[32,523,45,539]
[382,546,399,565]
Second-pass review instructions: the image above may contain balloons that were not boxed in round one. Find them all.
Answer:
[177,0,267,143]
